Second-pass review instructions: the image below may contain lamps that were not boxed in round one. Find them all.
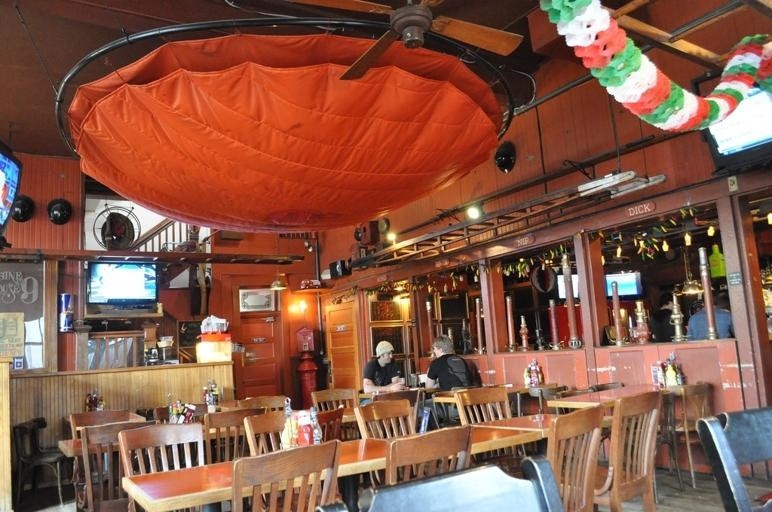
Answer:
[270,232,287,292]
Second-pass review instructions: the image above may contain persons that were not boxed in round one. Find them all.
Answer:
[416,334,476,423]
[650,290,687,343]
[361,339,409,395]
[681,290,735,341]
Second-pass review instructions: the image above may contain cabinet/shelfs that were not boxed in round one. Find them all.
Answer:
[708,244,726,277]
[281,405,324,451]
[168,383,219,424]
[658,353,684,389]
[85,388,107,414]
[530,366,539,387]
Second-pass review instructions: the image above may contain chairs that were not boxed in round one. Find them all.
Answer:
[695,407,771,509]
[10,417,72,510]
[59,376,716,512]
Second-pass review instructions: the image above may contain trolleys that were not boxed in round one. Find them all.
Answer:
[274,0,525,84]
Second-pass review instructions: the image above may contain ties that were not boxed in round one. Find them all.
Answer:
[0,144,21,231]
[605,273,641,297]
[82,259,158,304]
[703,87,772,169]
[557,275,580,301]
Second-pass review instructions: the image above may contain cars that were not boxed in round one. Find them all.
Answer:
[397,378,406,391]
[637,323,648,343]
[159,335,173,342]
[409,374,417,388]
[58,292,74,332]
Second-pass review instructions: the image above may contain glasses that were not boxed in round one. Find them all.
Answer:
[270,232,287,292]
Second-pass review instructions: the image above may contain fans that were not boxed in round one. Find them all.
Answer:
[274,0,525,84]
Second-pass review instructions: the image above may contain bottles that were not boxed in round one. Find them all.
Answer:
[376,340,395,358]
